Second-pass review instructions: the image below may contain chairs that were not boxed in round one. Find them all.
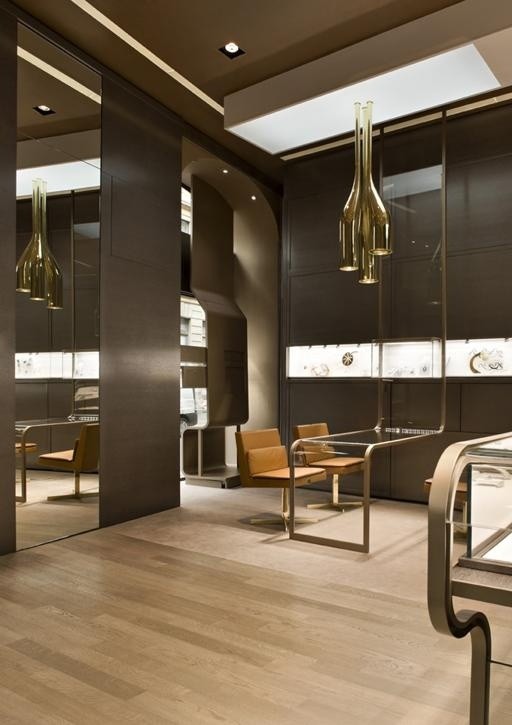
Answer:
[14,443,40,506]
[422,478,469,535]
[234,428,327,532]
[295,423,365,514]
[37,423,102,500]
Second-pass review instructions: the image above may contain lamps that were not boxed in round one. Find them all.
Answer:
[14,174,65,310]
[337,100,393,287]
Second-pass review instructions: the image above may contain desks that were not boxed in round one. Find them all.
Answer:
[287,427,436,553]
[14,420,92,502]
